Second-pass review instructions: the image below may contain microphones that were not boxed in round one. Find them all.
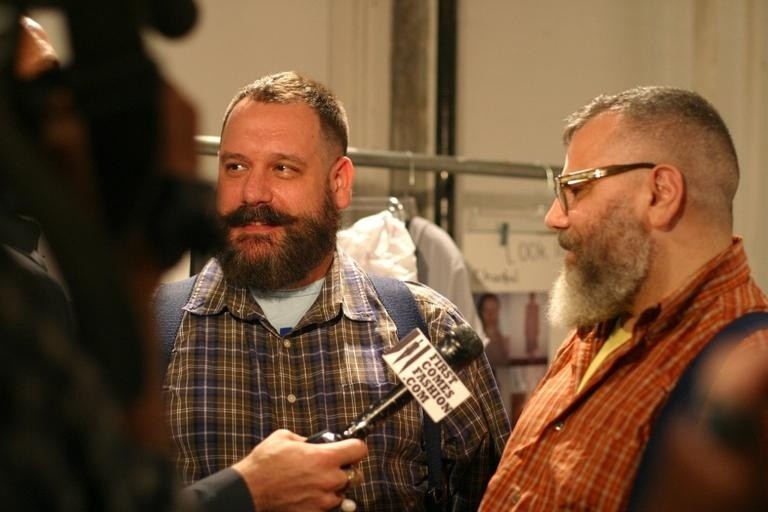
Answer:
[339,323,484,441]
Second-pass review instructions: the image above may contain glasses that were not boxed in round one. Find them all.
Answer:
[543,156,654,216]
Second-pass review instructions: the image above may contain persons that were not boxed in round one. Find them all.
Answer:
[0,7,202,511]
[162,416,371,512]
[140,70,513,512]
[522,292,541,360]
[478,292,511,364]
[476,81,766,511]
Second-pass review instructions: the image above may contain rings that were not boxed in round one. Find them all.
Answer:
[344,471,357,490]
